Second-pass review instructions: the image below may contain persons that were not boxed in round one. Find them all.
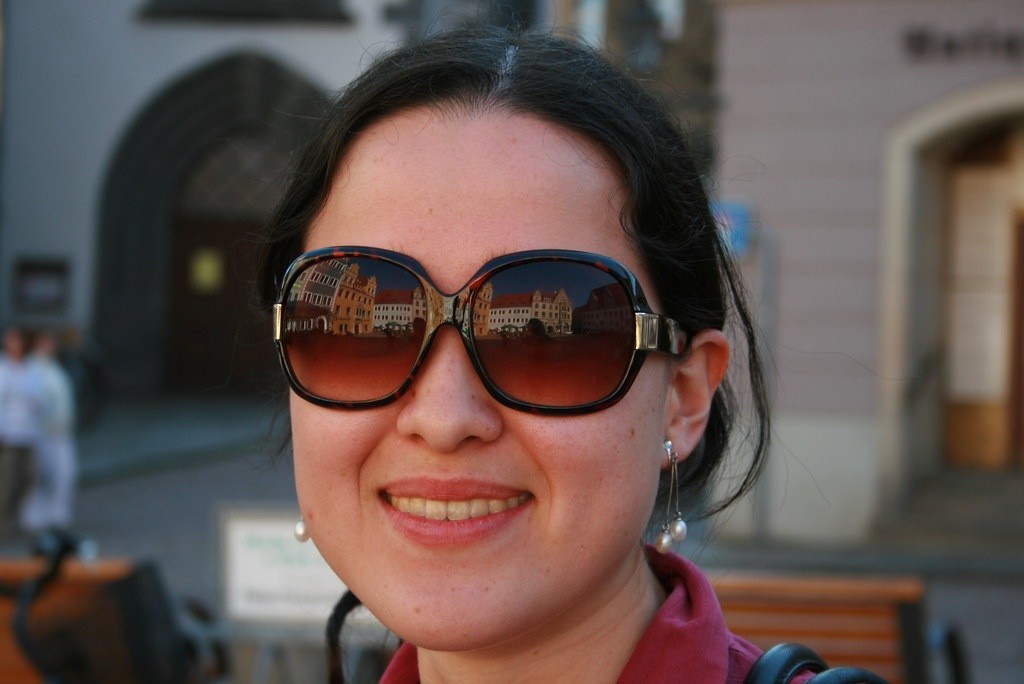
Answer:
[1,309,77,567]
[254,23,882,684]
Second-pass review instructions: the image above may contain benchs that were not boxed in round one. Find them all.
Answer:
[0,557,234,684]
[328,575,976,684]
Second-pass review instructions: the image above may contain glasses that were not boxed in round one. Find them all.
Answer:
[273,245,688,417]
